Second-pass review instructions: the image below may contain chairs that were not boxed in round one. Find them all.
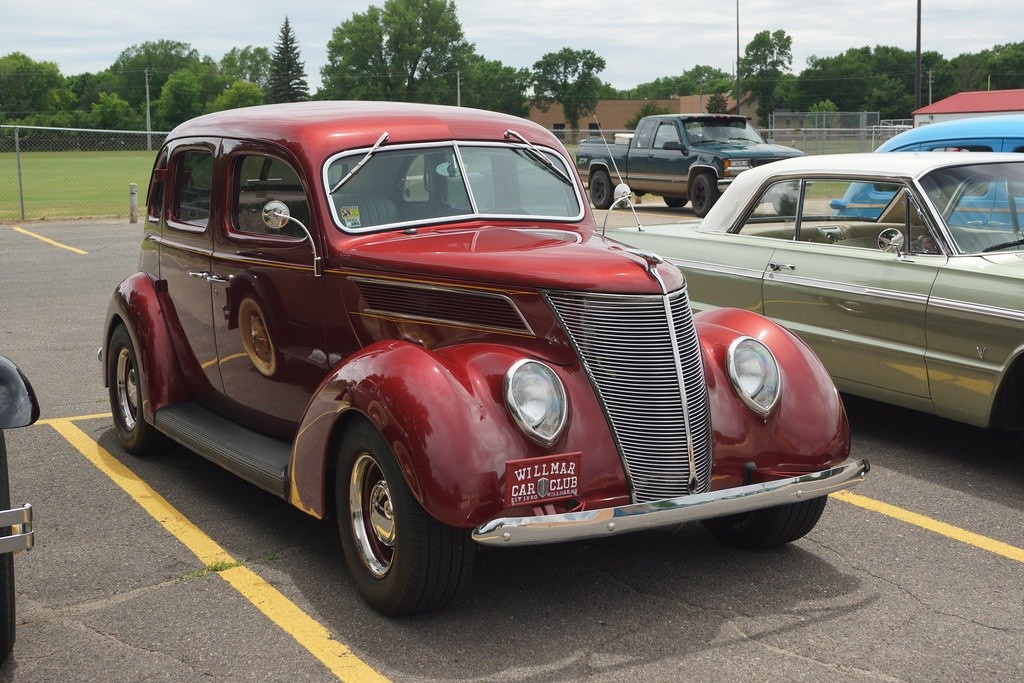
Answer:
[750,221,987,250]
[702,126,723,141]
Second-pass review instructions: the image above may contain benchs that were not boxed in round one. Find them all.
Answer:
[188,193,403,239]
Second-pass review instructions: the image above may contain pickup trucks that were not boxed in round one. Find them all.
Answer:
[576,113,813,217]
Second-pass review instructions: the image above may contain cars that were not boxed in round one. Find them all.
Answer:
[599,152,1023,467]
[830,115,1023,231]
[96,98,873,620]
